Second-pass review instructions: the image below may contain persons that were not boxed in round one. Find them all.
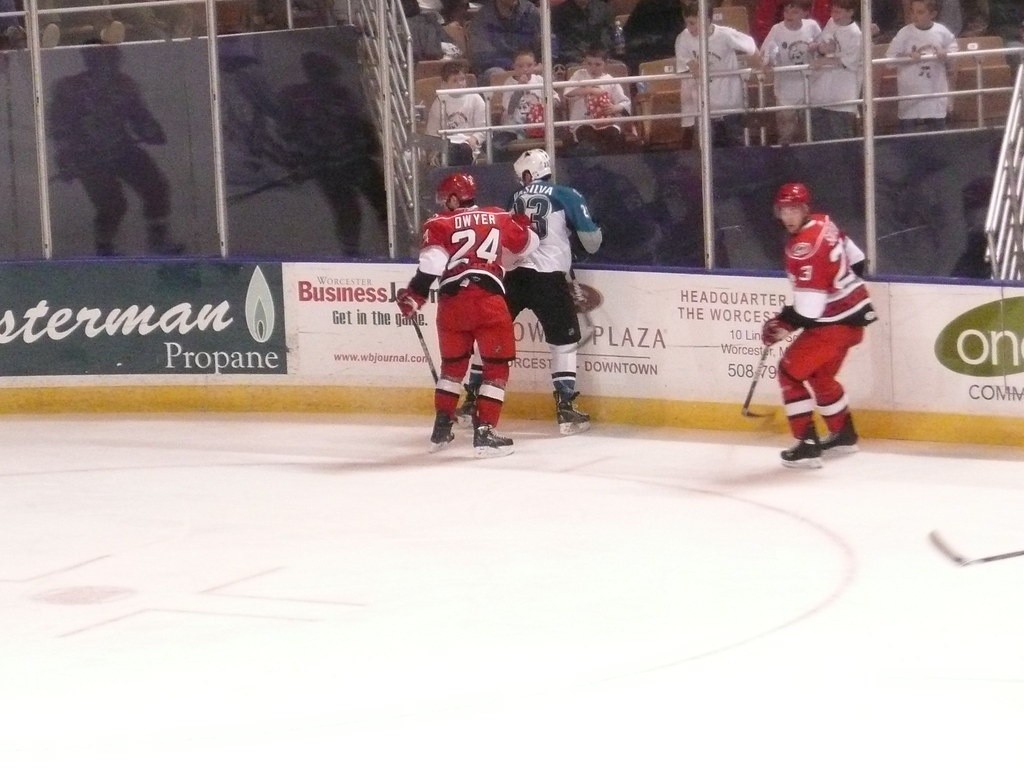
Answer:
[567,143,996,282]
[674,0,763,148]
[454,148,602,436]
[811,0,864,143]
[396,170,541,458]
[762,183,878,462]
[425,58,488,167]
[483,46,561,162]
[465,0,555,88]
[246,51,412,256]
[760,0,880,145]
[551,0,628,65]
[401,0,473,62]
[0,0,336,52]
[885,0,961,134]
[46,38,185,260]
[562,45,632,156]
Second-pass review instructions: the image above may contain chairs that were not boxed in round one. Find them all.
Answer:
[490,67,565,153]
[637,57,686,144]
[736,54,779,146]
[567,63,638,146]
[710,6,750,36]
[441,26,468,57]
[946,36,1013,130]
[415,73,487,171]
[871,43,904,136]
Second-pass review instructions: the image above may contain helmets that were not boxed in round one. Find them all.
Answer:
[434,173,476,205]
[772,183,810,218]
[512,148,553,187]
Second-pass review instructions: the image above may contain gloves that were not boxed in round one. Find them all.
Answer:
[512,213,532,227]
[762,315,796,346]
[396,285,426,320]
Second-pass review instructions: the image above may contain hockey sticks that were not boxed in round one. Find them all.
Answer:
[411,316,475,423]
[563,268,595,354]
[740,346,775,419]
[928,531,1024,568]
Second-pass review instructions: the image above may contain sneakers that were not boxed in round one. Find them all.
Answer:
[780,420,823,470]
[426,410,455,452]
[552,389,591,435]
[470,415,514,458]
[456,383,479,428]
[818,412,859,458]
